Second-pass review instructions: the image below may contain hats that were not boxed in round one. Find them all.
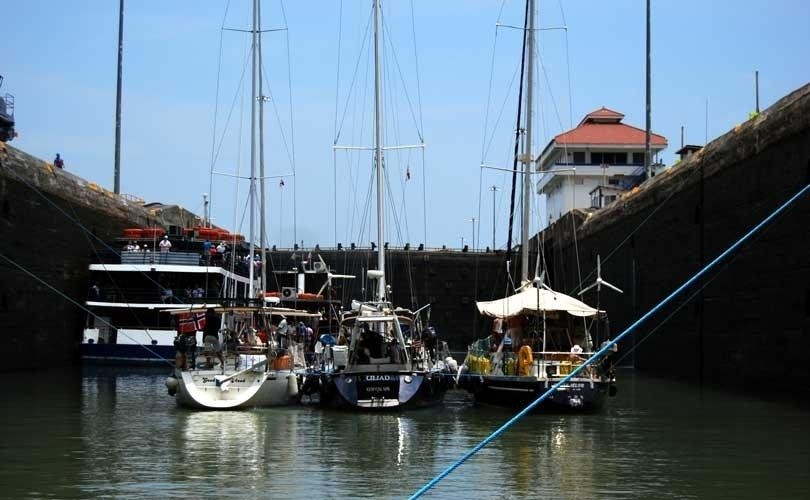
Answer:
[571,345,583,353]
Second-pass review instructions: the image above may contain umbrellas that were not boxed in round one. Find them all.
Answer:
[474,284,606,348]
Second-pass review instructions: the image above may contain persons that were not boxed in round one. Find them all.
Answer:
[141,242,151,256]
[203,239,262,279]
[54,152,65,170]
[123,239,134,253]
[132,240,140,254]
[492,317,508,350]
[274,313,314,353]
[568,345,588,363]
[158,235,172,265]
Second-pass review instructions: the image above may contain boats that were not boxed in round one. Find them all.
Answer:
[75,193,271,376]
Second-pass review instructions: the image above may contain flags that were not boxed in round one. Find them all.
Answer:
[177,311,206,334]
